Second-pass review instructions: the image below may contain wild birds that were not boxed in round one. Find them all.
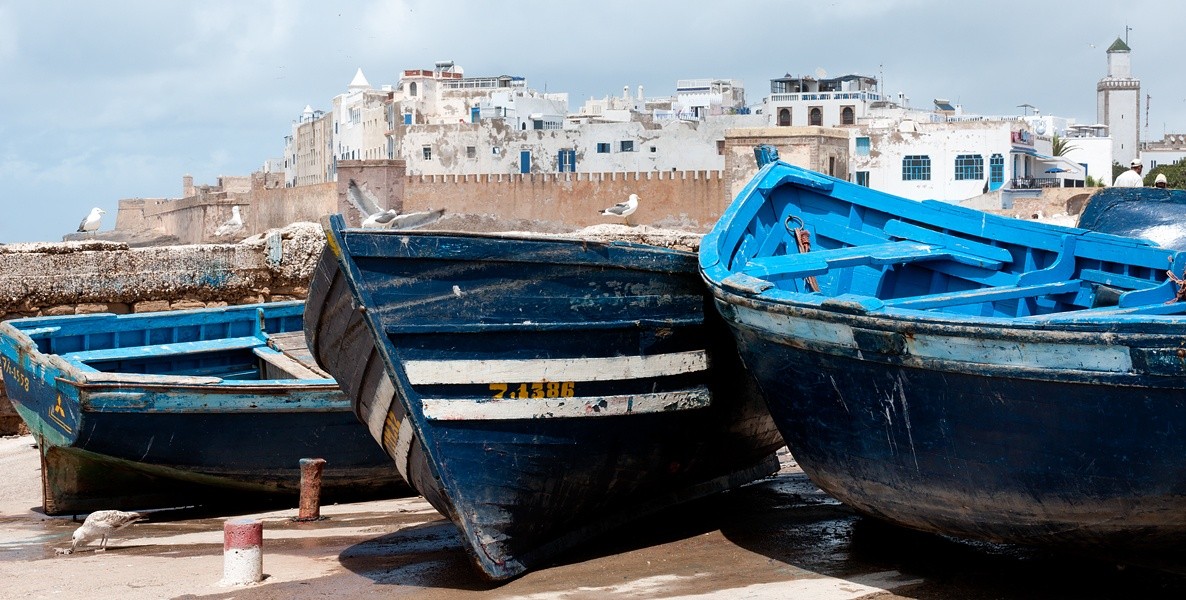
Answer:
[77,207,105,240]
[70,510,150,553]
[598,194,642,227]
[209,206,244,246]
[348,178,447,232]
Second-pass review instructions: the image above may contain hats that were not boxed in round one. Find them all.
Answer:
[1131,159,1143,167]
[1155,174,1166,184]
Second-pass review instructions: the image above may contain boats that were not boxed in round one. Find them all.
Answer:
[0,298,419,519]
[693,140,1186,591]
[1074,185,1186,251]
[301,212,795,584]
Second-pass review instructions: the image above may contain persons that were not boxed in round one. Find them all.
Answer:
[1154,173,1167,188]
[1017,175,1035,189]
[1114,159,1144,186]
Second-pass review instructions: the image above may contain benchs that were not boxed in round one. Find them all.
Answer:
[66,333,266,375]
[879,278,1082,310]
[742,239,1002,280]
[1035,298,1186,320]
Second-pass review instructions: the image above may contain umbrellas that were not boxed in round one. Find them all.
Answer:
[1016,104,1035,115]
[1045,168,1067,178]
[1062,123,1109,137]
